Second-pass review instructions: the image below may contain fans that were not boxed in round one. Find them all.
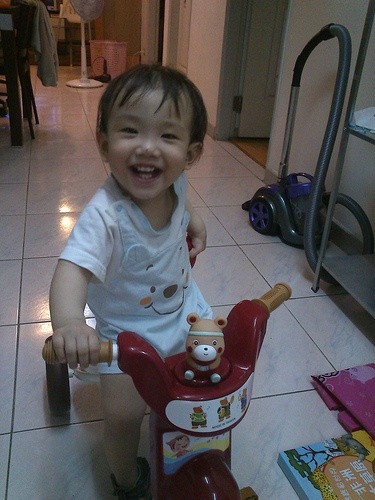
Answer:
[65,0,103,89]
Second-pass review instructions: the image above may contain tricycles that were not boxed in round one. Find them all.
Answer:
[43,281,295,500]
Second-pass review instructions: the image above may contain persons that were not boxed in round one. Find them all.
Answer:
[48,64,208,500]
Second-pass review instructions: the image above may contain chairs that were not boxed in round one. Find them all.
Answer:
[5,5,39,140]
[59,5,95,66]
[0,8,25,147]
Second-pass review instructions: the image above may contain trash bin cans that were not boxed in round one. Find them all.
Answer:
[89,38,128,83]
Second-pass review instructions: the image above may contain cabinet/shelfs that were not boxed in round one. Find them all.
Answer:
[305,0,375,318]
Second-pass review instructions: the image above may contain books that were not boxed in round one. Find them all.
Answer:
[277,429,375,500]
